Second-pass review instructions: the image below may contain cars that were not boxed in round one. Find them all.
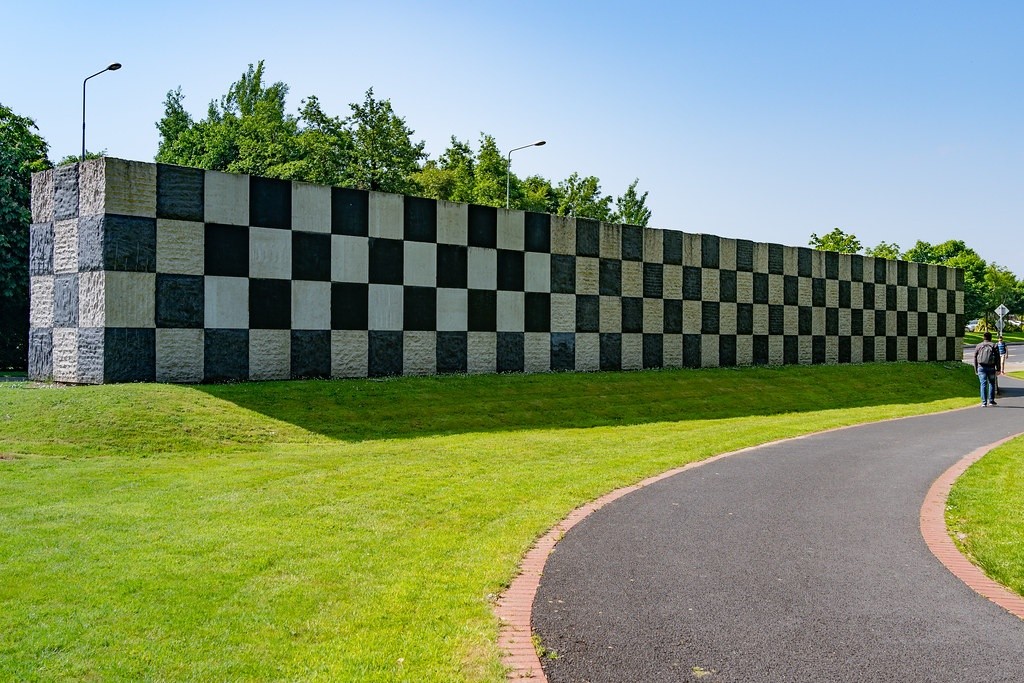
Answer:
[965,320,980,332]
[1007,320,1024,328]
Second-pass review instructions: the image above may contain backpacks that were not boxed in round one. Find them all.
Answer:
[976,342,997,365]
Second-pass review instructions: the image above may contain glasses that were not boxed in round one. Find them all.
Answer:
[999,340,1002,341]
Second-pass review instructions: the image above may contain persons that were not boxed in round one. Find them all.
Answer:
[974,332,1001,407]
[996,336,1008,375]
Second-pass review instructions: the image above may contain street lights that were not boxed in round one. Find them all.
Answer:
[507,141,547,209]
[82,63,122,161]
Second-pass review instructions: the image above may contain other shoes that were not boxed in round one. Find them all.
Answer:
[982,403,987,408]
[989,402,997,406]
[1001,372,1004,374]
